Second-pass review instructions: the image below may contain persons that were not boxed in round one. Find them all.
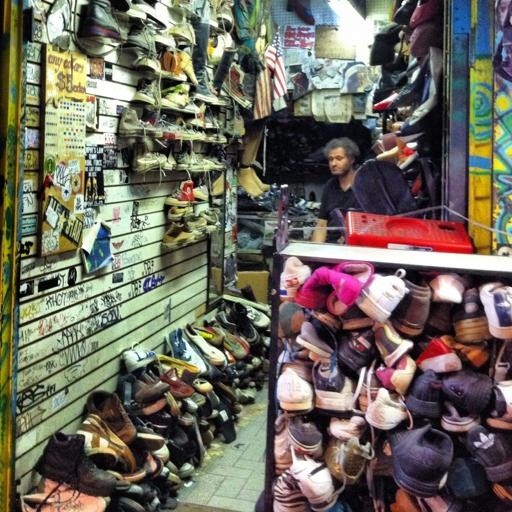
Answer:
[310,137,362,244]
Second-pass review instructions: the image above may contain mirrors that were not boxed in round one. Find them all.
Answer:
[205,154,227,312]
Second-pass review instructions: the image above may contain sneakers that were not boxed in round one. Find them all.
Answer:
[118,1,271,249]
[80,2,127,41]
[273,255,512,512]
[76,296,271,512]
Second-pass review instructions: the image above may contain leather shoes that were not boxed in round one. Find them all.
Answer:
[374,0,441,134]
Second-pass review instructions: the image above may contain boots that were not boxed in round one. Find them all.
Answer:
[34,432,117,498]
